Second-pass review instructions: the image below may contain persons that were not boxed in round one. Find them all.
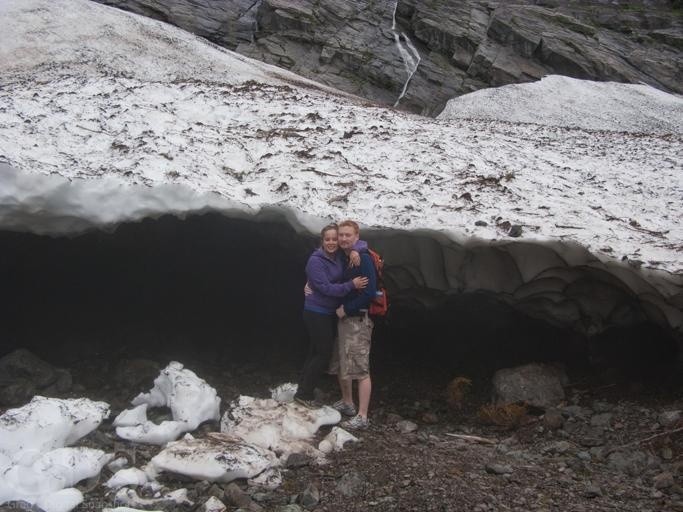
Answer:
[304,219,381,430]
[294,223,369,409]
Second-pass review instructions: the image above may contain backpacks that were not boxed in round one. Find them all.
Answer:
[368,248,391,317]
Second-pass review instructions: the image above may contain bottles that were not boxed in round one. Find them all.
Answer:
[376,291,384,303]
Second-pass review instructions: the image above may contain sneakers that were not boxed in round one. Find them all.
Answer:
[332,399,370,432]
[293,387,330,410]
